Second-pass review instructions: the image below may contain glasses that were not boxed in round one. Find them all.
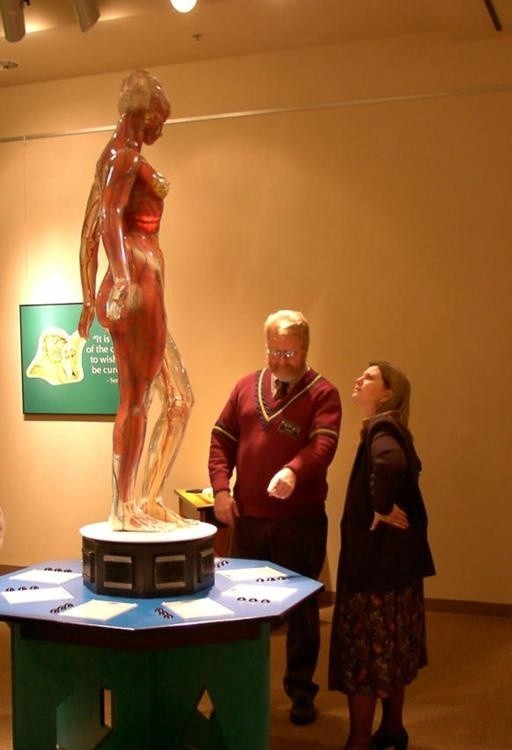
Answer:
[267,350,296,357]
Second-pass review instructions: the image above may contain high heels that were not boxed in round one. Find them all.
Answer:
[370,728,409,750]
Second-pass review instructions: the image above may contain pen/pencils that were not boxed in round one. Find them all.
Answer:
[198,493,212,504]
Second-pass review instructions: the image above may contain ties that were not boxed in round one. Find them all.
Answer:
[273,380,289,401]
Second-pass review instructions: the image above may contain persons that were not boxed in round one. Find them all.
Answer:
[78,69,196,530]
[208,309,342,726]
[328,359,437,750]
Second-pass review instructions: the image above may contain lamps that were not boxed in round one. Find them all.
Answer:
[73,1,101,33]
[1,0,25,46]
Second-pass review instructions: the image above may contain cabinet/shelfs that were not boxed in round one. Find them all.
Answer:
[173,486,237,558]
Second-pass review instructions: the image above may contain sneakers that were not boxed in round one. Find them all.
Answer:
[290,695,315,725]
